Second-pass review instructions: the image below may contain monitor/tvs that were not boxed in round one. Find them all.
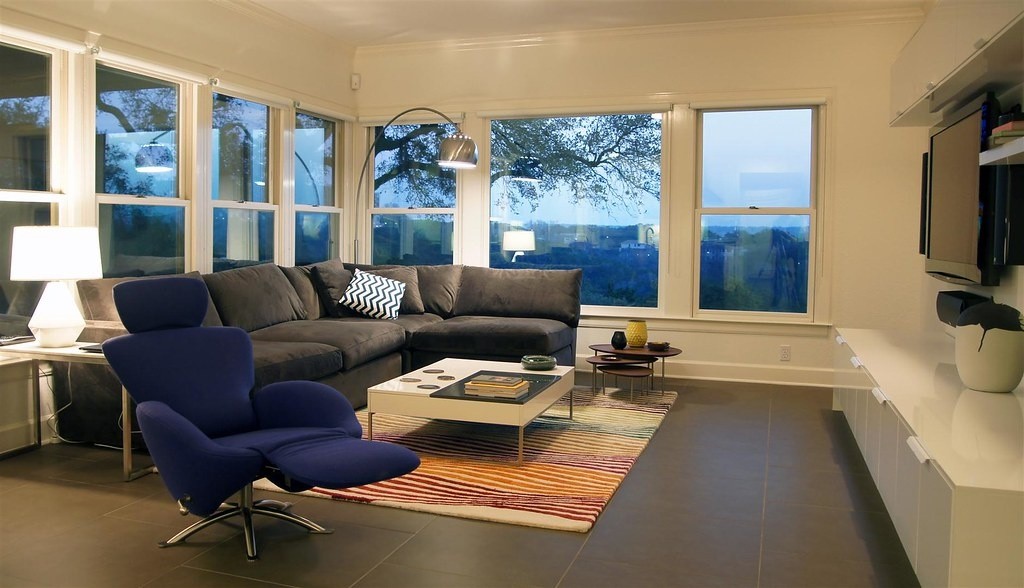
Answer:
[923,88,1005,288]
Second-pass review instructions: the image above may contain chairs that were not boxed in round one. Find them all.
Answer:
[103,279,420,561]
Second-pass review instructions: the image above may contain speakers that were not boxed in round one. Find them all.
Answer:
[919,151,928,257]
[936,290,991,328]
[990,105,1024,266]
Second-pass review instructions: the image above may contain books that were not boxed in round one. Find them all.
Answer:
[464,374,530,399]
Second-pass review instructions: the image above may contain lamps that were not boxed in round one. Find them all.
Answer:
[504,231,535,259]
[11,226,103,349]
[135,138,175,173]
[352,108,478,264]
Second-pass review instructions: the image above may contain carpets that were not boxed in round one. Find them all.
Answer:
[253,385,678,533]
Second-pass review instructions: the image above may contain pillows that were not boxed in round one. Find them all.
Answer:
[312,267,356,317]
[362,266,425,314]
[337,268,405,319]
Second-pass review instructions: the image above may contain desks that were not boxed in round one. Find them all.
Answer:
[587,341,681,401]
[0,342,156,481]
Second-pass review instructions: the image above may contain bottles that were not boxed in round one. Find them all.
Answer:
[611,331,627,350]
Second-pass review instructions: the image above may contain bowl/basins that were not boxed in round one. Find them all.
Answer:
[648,342,671,350]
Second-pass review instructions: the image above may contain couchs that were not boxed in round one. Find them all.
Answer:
[55,258,583,453]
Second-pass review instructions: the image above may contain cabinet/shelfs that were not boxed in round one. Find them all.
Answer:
[833,329,1024,588]
[887,0,1024,165]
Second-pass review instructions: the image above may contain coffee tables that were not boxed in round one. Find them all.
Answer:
[368,357,575,463]
